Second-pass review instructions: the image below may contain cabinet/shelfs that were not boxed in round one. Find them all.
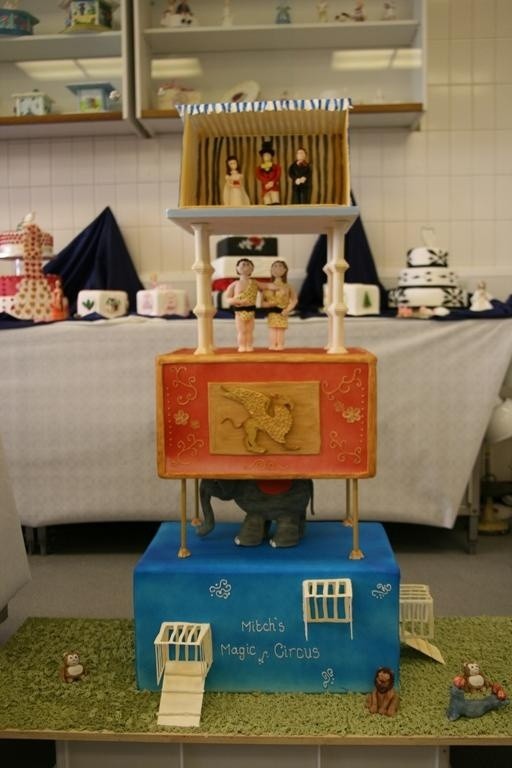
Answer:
[132,0,427,135]
[0,0,147,144]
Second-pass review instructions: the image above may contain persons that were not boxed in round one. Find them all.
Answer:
[220,257,280,352]
[289,147,314,204]
[222,154,254,206]
[255,139,282,204]
[260,260,300,350]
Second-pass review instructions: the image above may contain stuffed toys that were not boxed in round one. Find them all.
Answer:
[62,649,89,684]
[361,663,404,721]
[447,664,509,723]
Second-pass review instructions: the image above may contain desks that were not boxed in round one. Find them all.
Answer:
[0,317,511,553]
